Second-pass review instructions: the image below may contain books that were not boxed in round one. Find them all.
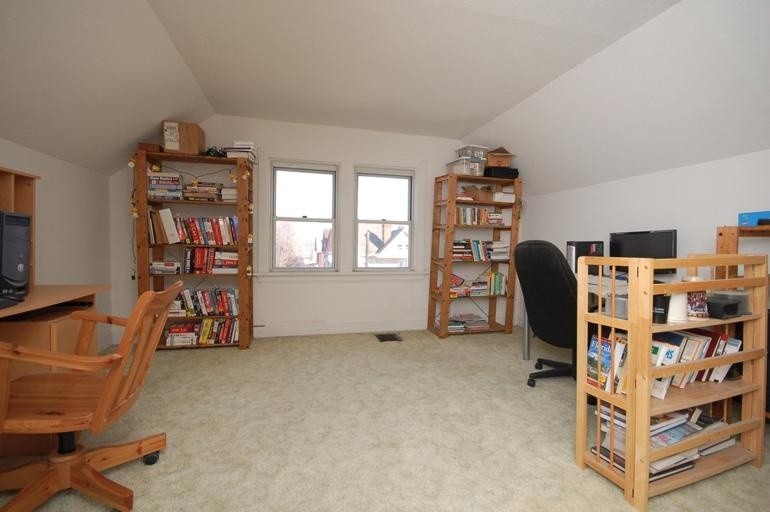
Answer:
[584,327,743,482]
[435,183,517,335]
[224,141,257,164]
[147,161,242,347]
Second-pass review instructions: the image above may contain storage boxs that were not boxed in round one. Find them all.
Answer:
[161,119,207,156]
[445,144,513,176]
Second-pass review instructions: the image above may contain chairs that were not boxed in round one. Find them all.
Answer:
[513,240,605,405]
[0,279,184,512]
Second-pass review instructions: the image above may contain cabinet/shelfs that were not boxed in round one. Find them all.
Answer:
[576,252,769,511]
[427,174,524,338]
[131,145,254,350]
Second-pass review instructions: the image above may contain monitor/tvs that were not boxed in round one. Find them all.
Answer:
[567,241,604,276]
[609,229,677,284]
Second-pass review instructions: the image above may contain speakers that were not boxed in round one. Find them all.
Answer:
[0,211,32,309]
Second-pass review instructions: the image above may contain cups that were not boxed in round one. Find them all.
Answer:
[667,275,710,323]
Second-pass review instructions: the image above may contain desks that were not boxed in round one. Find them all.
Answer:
[1,166,110,458]
